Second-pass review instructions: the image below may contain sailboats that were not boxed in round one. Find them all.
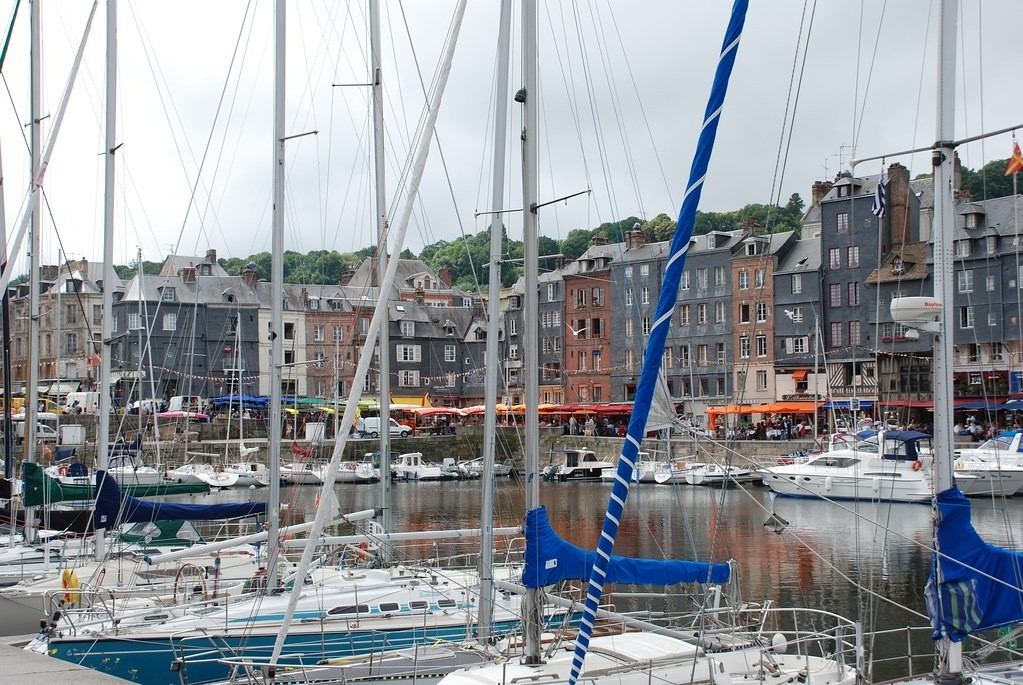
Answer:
[0,0,1022,684]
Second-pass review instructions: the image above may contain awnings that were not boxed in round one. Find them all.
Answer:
[875,399,934,407]
[954,396,1007,405]
[792,371,807,381]
[823,401,876,409]
[954,400,1023,410]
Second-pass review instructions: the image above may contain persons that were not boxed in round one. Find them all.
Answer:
[954,411,1023,443]
[715,414,806,440]
[62,397,171,415]
[415,414,628,436]
[903,416,933,433]
[859,411,873,428]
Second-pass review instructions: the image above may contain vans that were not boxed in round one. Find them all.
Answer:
[17,422,59,444]
[364,417,413,438]
[0,397,66,413]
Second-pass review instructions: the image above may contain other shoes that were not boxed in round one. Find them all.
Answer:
[781,438,788,441]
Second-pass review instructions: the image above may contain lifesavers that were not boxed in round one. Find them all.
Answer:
[58,466,69,475]
[60,569,79,601]
[913,459,922,471]
[214,465,222,474]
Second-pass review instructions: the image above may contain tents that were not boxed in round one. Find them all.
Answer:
[403,402,633,415]
[704,402,825,430]
[206,391,431,416]
